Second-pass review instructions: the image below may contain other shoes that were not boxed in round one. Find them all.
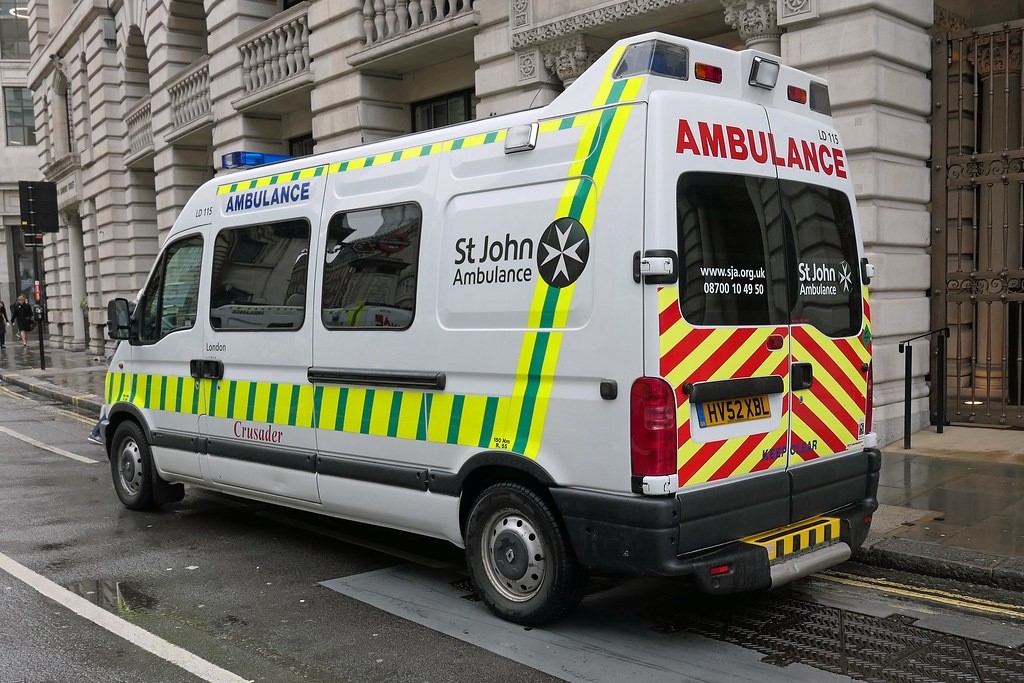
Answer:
[26,344,29,347]
[16,334,21,338]
[1,346,7,349]
[23,343,26,346]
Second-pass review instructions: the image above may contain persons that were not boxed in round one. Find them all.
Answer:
[0,294,33,348]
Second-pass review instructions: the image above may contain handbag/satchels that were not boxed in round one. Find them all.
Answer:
[30,320,36,331]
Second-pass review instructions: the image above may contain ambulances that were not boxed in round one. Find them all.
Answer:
[88,32,883,626]
